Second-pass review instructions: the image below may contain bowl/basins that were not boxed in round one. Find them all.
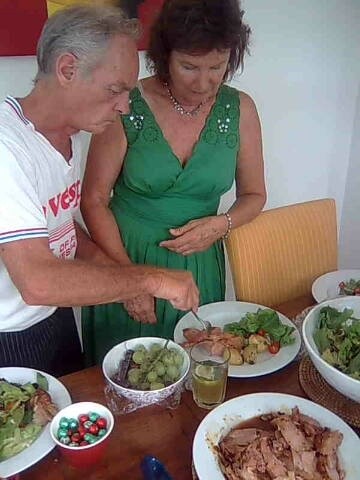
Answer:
[51,402,114,468]
[302,296,359,403]
[102,336,189,405]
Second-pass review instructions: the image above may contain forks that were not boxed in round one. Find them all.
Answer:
[192,308,211,335]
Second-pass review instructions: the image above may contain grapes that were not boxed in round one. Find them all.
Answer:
[128,336,184,391]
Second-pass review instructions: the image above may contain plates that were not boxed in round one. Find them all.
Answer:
[193,392,360,480]
[0,368,73,478]
[311,270,360,303]
[174,301,301,377]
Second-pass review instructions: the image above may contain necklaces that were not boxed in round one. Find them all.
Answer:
[158,78,217,122]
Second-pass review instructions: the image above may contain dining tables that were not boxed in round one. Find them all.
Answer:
[0,290,360,480]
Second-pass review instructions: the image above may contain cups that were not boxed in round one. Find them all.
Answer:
[190,340,231,410]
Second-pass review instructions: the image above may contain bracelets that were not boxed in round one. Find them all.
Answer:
[222,215,233,240]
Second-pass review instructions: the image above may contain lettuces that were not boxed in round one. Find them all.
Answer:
[339,278,360,295]
[312,304,360,381]
[223,307,296,347]
[0,371,59,462]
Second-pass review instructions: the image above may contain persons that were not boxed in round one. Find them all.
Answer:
[0,4,201,380]
[80,0,268,370]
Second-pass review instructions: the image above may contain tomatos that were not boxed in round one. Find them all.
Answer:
[256,328,280,354]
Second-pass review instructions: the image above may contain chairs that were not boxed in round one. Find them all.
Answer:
[224,198,338,307]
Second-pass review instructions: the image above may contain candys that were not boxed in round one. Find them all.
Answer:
[57,412,107,447]
